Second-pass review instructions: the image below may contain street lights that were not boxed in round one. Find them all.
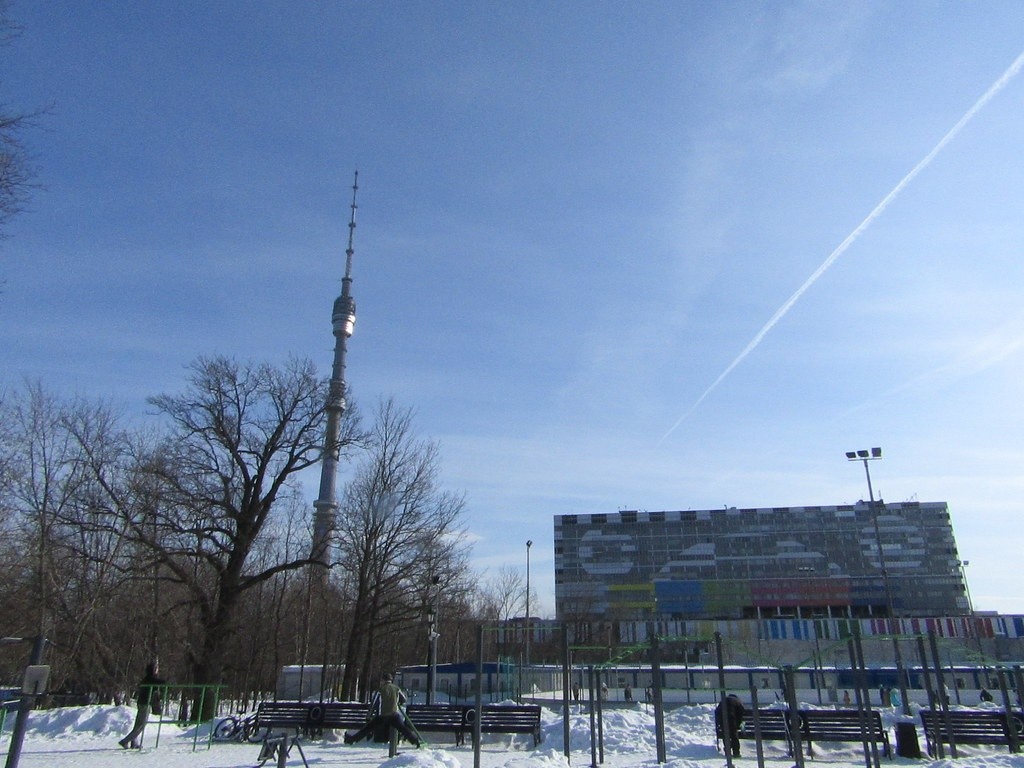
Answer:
[431,576,439,705]
[845,447,912,716]
[526,540,533,664]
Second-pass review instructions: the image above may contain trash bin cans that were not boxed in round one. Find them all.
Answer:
[894,722,921,758]
[879,687,891,707]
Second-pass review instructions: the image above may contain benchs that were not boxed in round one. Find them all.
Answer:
[716,709,892,760]
[253,702,379,742]
[918,710,1024,761]
[405,702,541,747]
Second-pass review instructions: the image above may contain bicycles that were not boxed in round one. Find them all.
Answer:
[213,710,273,744]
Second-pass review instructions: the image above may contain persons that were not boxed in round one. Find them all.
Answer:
[119,662,167,749]
[601,682,608,704]
[624,684,633,702]
[572,682,579,700]
[647,680,653,689]
[716,693,747,756]
[843,683,1021,707]
[177,696,189,727]
[344,674,421,747]
